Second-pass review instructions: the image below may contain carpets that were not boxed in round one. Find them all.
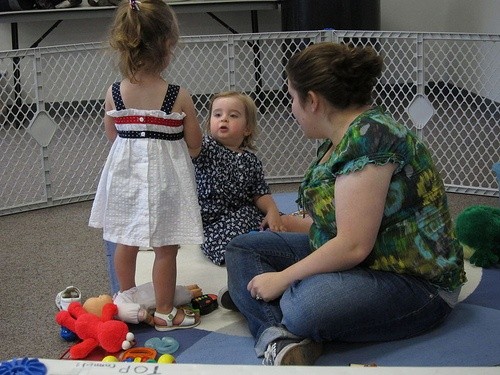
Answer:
[107,192,500,366]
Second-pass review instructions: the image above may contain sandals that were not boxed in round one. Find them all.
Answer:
[154,305,202,332]
[55,286,81,311]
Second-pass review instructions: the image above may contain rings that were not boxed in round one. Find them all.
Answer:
[256,294,262,299]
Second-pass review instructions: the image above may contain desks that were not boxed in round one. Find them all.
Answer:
[0,0,279,129]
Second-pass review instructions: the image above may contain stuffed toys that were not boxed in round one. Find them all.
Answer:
[453,205,500,268]
[55,302,136,360]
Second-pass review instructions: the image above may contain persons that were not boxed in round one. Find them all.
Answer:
[88,0,201,332]
[83,282,202,324]
[217,42,467,366]
[190,91,314,266]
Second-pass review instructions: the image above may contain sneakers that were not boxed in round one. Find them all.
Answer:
[217,286,236,311]
[263,337,323,366]
